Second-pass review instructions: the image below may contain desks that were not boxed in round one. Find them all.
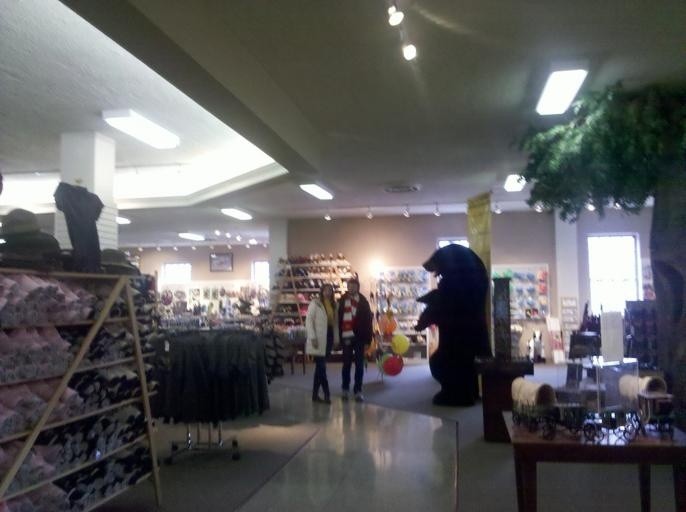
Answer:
[503,410,686,512]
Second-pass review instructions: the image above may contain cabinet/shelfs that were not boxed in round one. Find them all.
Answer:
[623,300,659,378]
[477,357,537,444]
[0,267,168,512]
[268,257,432,362]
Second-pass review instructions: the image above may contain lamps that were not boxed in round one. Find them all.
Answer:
[102,108,182,153]
[531,60,590,118]
[386,7,420,63]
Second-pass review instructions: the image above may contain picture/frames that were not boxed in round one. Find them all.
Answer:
[206,252,234,273]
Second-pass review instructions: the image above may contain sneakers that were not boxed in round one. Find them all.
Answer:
[312,395,330,403]
[342,390,364,401]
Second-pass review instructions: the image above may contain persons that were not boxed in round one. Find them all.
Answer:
[337,277,371,400]
[303,283,340,404]
